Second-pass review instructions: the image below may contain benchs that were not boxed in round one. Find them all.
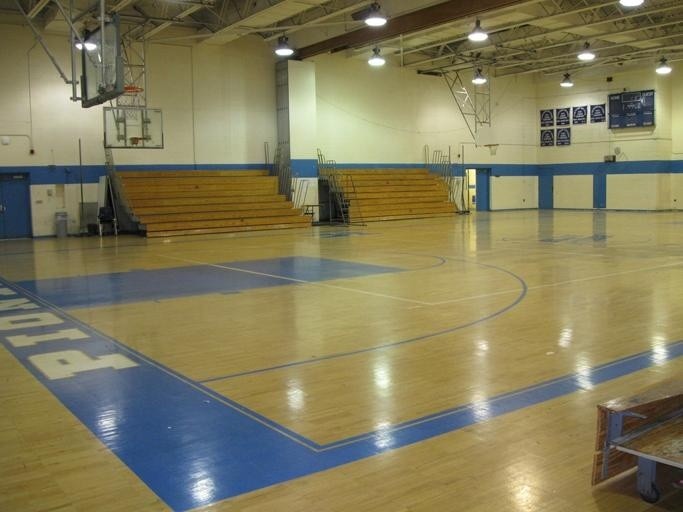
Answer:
[115,169,312,237]
[320,166,461,224]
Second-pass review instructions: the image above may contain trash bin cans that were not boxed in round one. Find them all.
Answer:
[55,212,67,238]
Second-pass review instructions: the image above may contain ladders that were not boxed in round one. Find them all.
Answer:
[328,174,364,227]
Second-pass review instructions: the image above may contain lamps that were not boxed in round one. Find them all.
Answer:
[365,1,386,28]
[471,69,486,85]
[271,36,294,57]
[468,16,488,44]
[576,41,595,62]
[366,46,385,69]
[654,56,671,74]
[75,33,99,53]
[560,72,572,88]
